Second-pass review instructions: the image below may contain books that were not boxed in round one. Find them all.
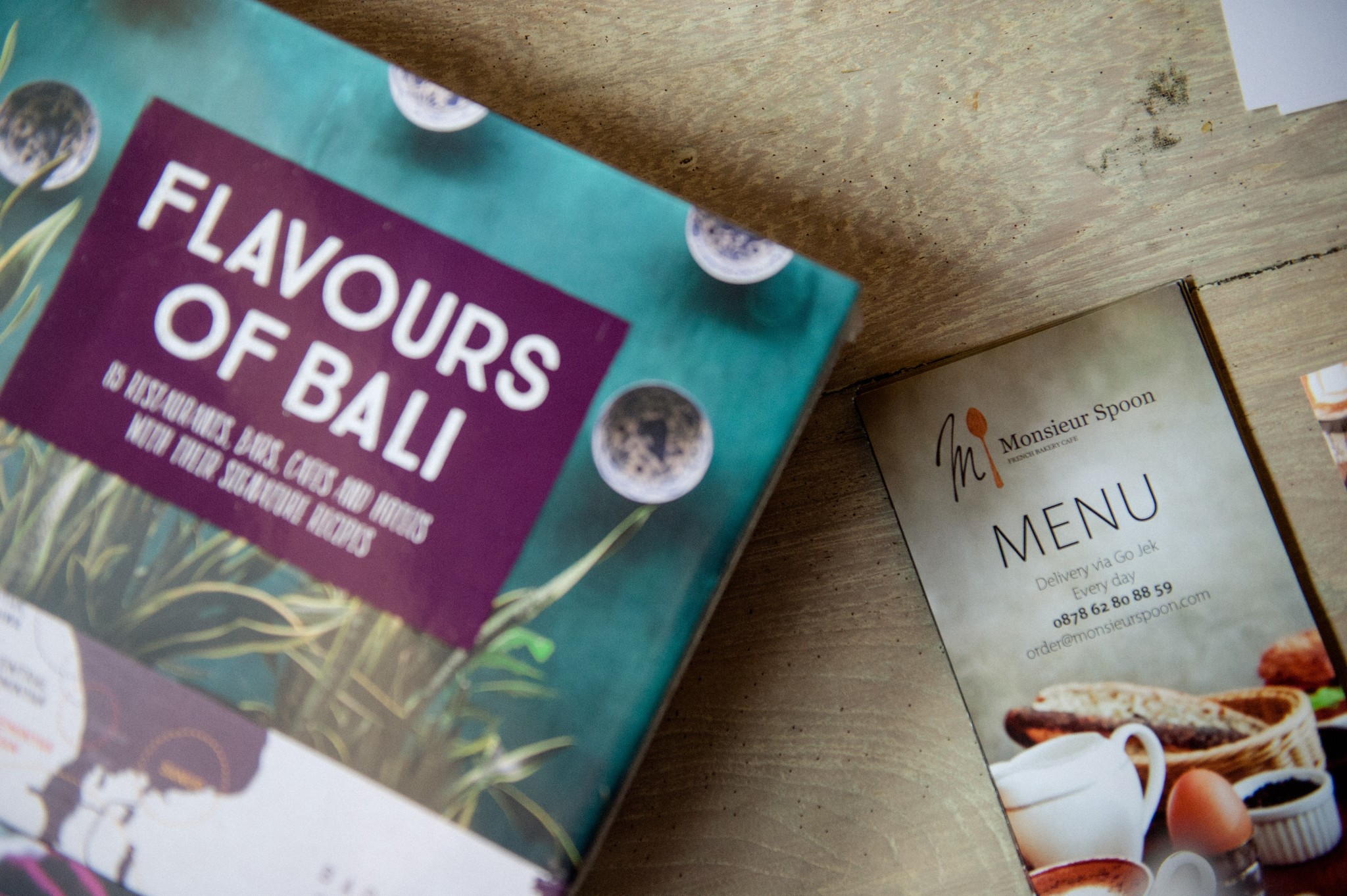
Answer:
[0,1,860,896]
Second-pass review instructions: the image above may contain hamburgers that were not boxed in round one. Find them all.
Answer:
[1257,632,1347,725]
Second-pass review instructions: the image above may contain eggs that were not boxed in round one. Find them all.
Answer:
[1164,767,1255,862]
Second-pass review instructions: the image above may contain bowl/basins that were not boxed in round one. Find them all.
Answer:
[1227,769,1341,867]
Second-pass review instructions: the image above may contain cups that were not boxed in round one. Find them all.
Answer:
[682,205,793,285]
[0,75,102,191]
[592,377,713,502]
[990,720,1164,865]
[387,66,489,132]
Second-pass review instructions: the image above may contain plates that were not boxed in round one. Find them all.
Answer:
[1028,856,1154,896]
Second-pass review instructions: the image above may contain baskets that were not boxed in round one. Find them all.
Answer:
[1130,686,1325,816]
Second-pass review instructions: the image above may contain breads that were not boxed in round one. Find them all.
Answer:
[1001,681,1271,758]
